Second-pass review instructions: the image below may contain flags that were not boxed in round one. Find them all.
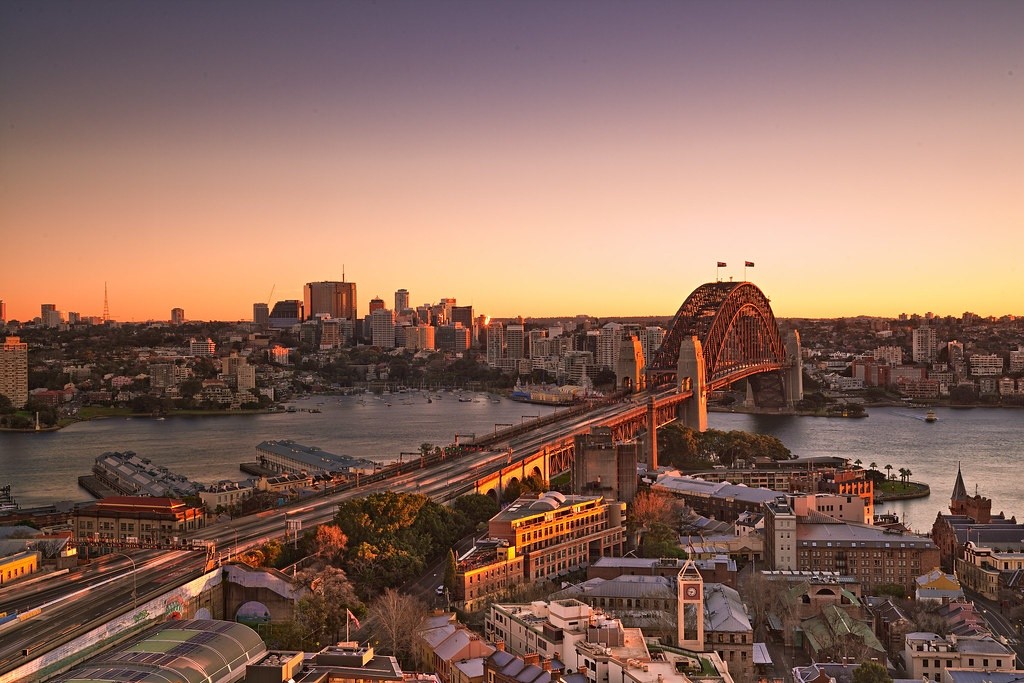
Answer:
[717,262,727,267]
[745,262,754,267]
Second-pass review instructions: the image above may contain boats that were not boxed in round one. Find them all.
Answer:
[926,408,938,423]
